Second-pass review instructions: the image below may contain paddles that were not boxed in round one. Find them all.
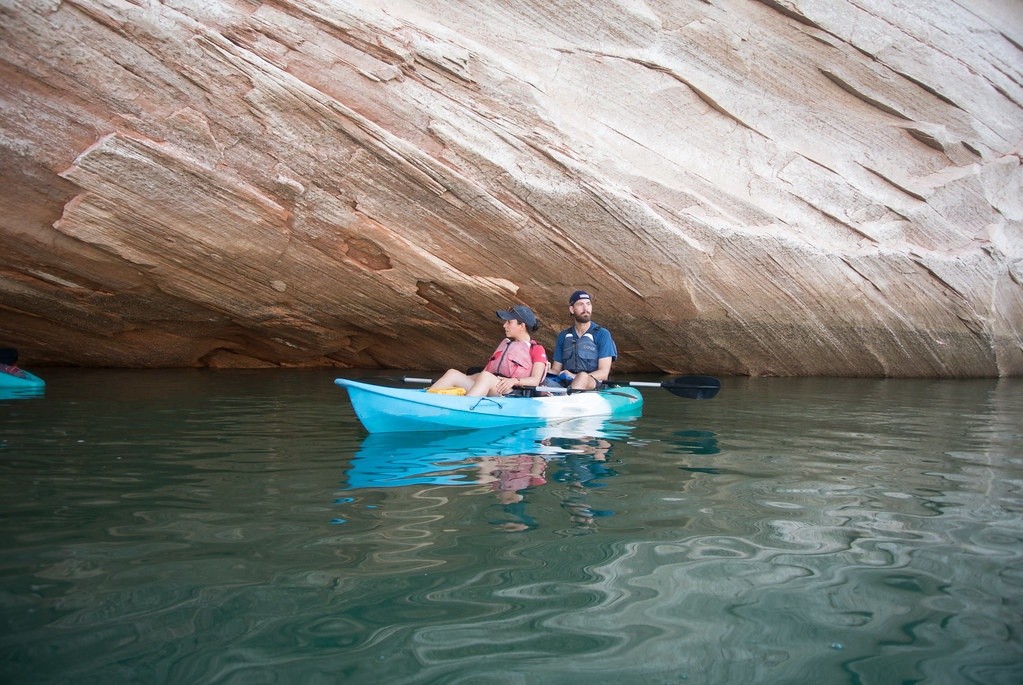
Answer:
[466,364,724,403]
[345,373,640,401]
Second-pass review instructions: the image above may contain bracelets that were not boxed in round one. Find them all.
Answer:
[515,377,521,386]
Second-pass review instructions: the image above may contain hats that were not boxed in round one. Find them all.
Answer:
[569,291,591,317]
[496,306,537,329]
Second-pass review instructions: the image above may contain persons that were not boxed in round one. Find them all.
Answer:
[428,306,547,397]
[541,291,618,396]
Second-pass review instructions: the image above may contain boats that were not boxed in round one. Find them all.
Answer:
[0,362,46,390]
[334,374,647,433]
[340,412,635,491]
[0,390,48,401]
